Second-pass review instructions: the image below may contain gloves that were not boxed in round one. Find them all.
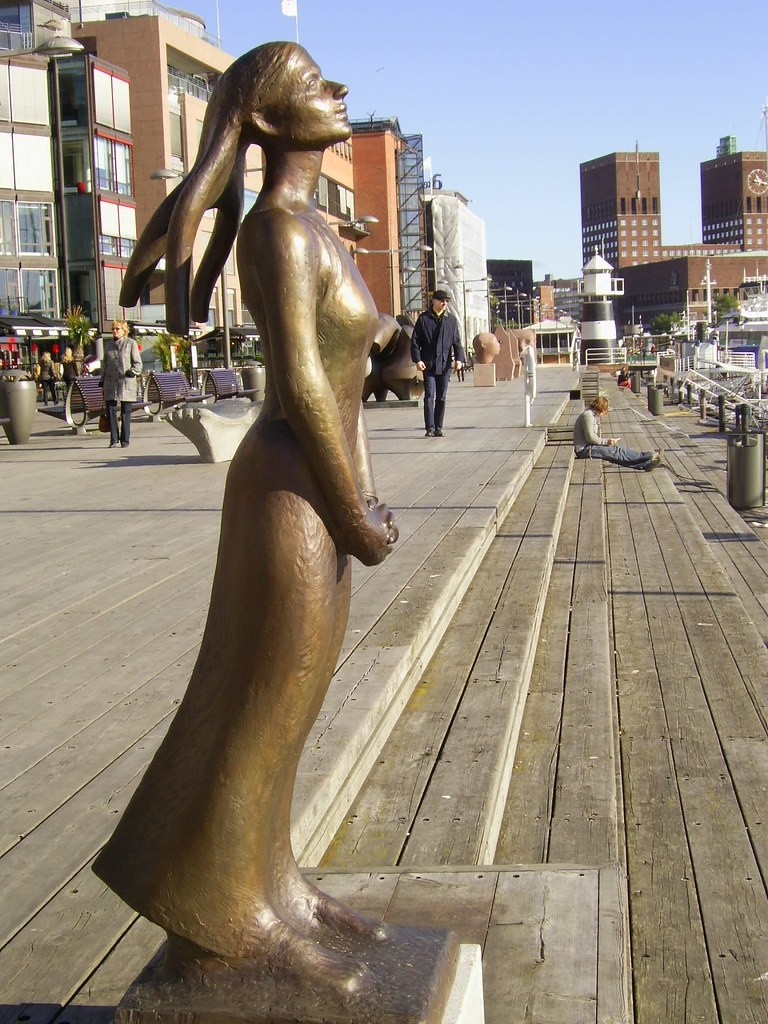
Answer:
[126,370,136,378]
[98,382,104,387]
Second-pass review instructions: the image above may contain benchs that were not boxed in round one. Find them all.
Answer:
[66,370,260,435]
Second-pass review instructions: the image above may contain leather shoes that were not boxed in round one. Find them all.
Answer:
[426,430,433,436]
[435,428,445,437]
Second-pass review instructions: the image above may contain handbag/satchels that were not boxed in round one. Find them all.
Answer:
[99,392,111,432]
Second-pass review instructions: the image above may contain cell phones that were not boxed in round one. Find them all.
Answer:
[616,438,620,441]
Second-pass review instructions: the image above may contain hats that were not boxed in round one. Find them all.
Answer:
[432,290,451,299]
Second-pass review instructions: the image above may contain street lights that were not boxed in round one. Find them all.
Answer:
[437,278,493,356]
[485,292,582,365]
[402,263,467,294]
[353,245,433,321]
[466,286,513,334]
[148,166,262,367]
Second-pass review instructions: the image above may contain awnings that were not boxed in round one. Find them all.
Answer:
[232,328,261,341]
[0,316,75,336]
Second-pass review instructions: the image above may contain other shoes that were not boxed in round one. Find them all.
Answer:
[644,448,663,472]
[109,443,116,448]
[121,443,128,448]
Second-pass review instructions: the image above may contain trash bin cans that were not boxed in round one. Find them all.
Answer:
[647,383,664,415]
[726,430,764,508]
[241,361,266,402]
[630,371,641,393]
[0,370,36,444]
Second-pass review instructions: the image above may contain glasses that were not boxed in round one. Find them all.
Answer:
[111,327,122,330]
[436,299,449,302]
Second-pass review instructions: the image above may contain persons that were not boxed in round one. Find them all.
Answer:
[90,41,399,1003]
[572,397,662,472]
[367,110,377,125]
[617,367,631,388]
[473,333,500,364]
[410,290,465,438]
[59,347,80,392]
[98,320,143,449]
[39,351,59,406]
